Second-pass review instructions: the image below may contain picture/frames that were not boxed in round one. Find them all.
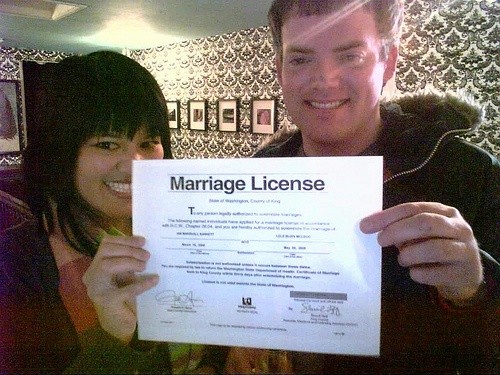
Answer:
[250,99,276,135]
[217,99,239,132]
[188,100,207,131]
[0,79,23,155]
[166,101,180,129]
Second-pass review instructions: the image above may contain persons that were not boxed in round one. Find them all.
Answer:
[0,50,294,375]
[225,1,499,375]
